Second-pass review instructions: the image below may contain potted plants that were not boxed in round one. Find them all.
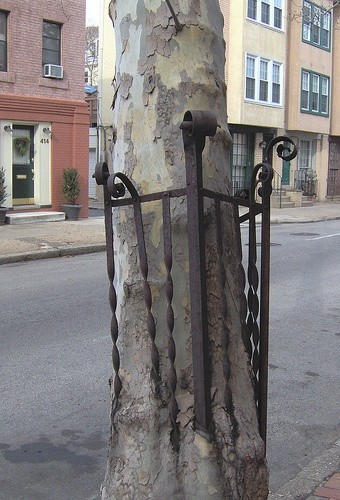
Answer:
[61,167,82,221]
[0,167,7,226]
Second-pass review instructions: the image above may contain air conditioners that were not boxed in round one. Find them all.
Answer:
[43,63,64,79]
